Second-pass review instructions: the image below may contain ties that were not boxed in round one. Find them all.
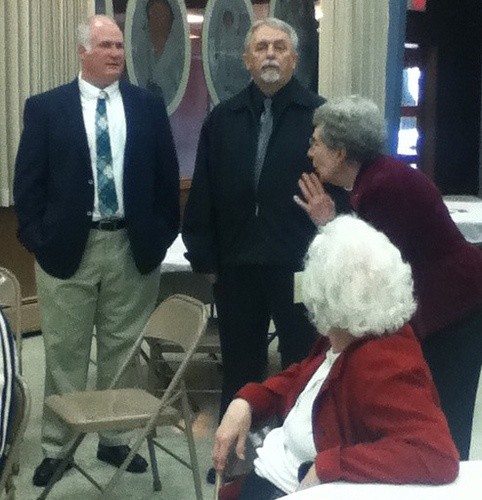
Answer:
[95,92,119,219]
[255,98,273,196]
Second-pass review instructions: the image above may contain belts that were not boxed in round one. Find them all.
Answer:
[92,220,126,231]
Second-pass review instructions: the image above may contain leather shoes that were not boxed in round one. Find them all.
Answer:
[96,442,149,474]
[206,465,219,483]
[33,455,73,488]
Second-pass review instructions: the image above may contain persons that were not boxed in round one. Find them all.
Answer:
[211,214,459,500]
[294,96,482,462]
[179,17,354,485]
[13,15,179,487]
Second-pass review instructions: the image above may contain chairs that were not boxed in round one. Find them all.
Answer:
[44,293,209,500]
[147,271,223,393]
[0,265,32,500]
